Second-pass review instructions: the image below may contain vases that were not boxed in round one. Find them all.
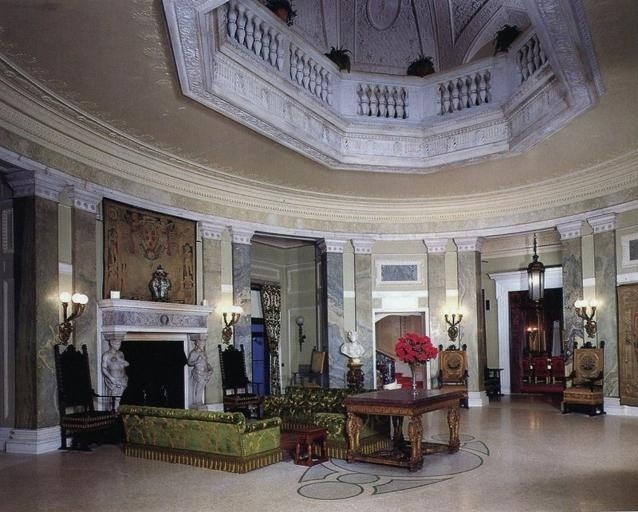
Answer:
[410,367,418,395]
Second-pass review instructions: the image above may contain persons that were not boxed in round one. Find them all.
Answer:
[102,339,131,413]
[187,339,214,406]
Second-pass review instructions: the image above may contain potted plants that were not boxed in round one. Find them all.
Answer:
[493,25,522,56]
[266,0,297,26]
[407,55,434,77]
[324,46,352,73]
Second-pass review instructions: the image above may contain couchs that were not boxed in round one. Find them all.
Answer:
[265,386,391,458]
[117,405,284,472]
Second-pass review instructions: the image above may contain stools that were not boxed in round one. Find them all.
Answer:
[294,425,328,467]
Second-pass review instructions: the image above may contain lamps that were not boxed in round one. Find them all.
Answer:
[295,316,308,351]
[574,299,598,338]
[442,305,466,339]
[56,291,88,340]
[528,233,544,308]
[222,304,243,342]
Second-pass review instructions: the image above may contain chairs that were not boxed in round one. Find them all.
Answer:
[437,344,469,409]
[55,344,120,451]
[286,345,325,392]
[555,341,605,416]
[484,366,504,402]
[218,344,265,421]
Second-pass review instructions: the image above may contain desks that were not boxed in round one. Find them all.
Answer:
[344,387,467,473]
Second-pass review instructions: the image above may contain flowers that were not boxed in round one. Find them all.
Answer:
[395,332,438,374]
